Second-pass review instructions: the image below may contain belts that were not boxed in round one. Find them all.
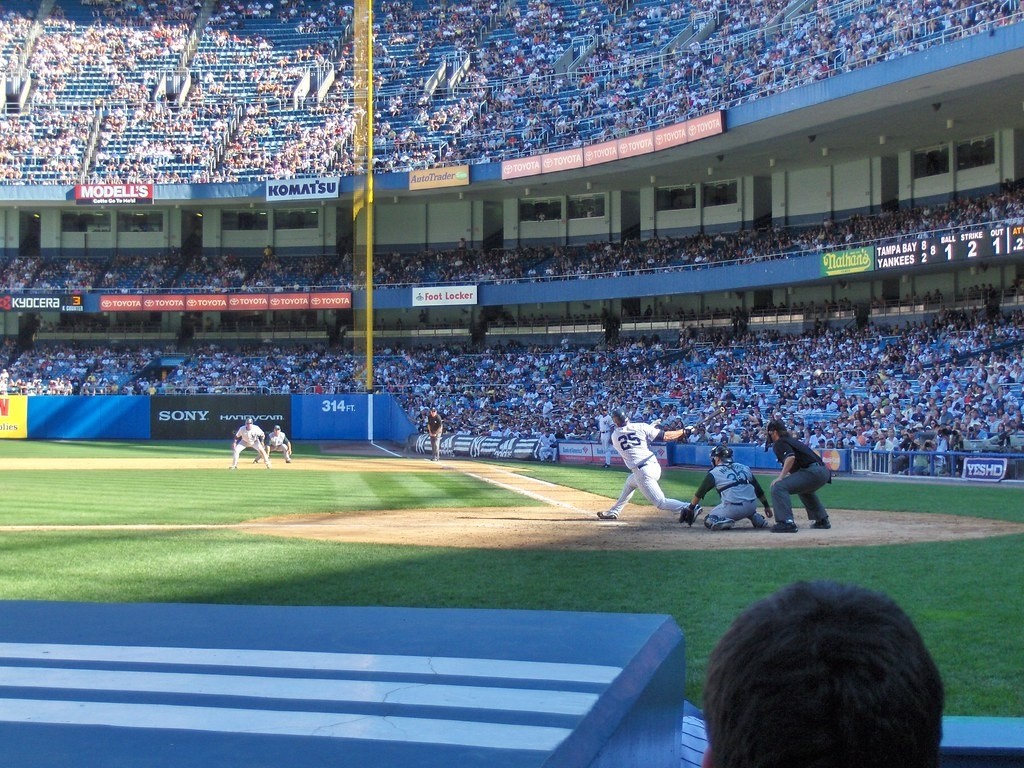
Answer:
[638,463,647,469]
[731,499,756,505]
[802,462,825,468]
[602,431,608,433]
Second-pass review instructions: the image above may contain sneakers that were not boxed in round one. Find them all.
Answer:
[693,504,703,521]
[809,517,831,529]
[758,519,768,528]
[597,511,618,519]
[770,518,799,533]
[712,518,735,530]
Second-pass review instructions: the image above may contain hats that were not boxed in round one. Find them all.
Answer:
[274,425,281,430]
[431,407,436,411]
[246,418,253,424]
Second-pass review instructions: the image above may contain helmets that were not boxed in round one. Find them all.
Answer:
[709,445,734,467]
[611,409,628,427]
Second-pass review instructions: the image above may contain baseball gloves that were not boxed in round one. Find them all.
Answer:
[678,503,695,528]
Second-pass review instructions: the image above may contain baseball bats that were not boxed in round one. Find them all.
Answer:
[693,406,727,426]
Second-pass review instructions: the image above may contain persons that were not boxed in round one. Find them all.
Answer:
[680,445,773,531]
[0,0,1024,481]
[700,577,945,768]
[229,418,272,469]
[597,409,703,522]
[768,419,831,533]
[253,425,292,463]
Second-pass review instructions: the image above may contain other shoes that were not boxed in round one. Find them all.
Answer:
[286,460,292,463]
[431,458,439,461]
[603,463,610,468]
[266,464,271,469]
[229,464,238,469]
[253,460,258,464]
